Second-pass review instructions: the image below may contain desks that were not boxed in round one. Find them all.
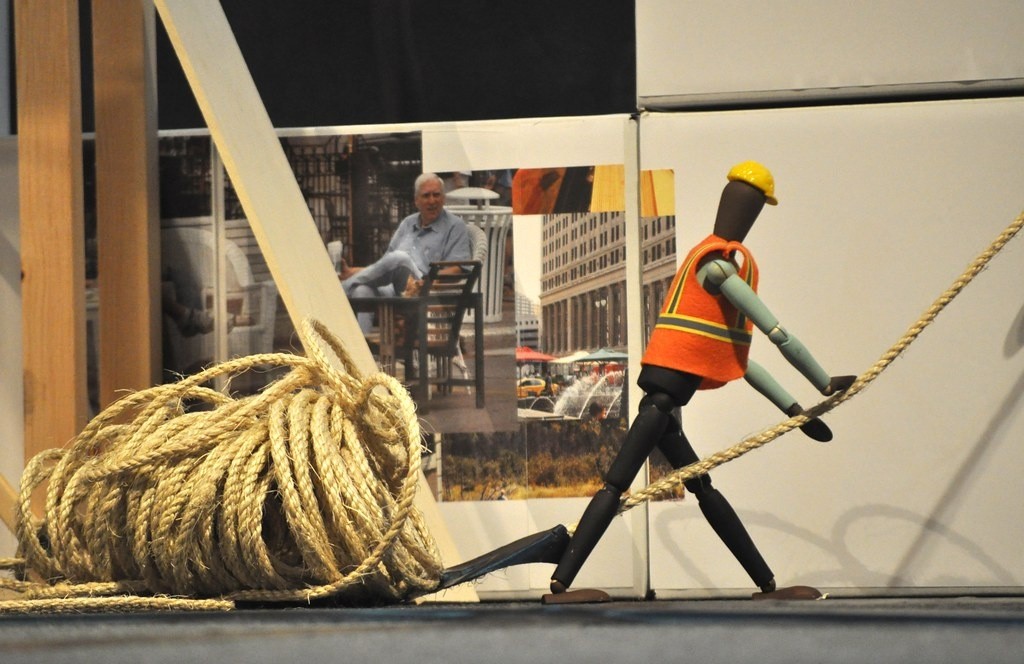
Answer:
[347,294,484,412]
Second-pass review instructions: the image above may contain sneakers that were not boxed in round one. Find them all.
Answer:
[174,307,214,337]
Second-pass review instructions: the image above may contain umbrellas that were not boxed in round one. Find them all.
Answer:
[516,346,629,398]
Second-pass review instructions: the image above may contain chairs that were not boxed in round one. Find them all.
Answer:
[161,228,277,388]
[366,260,484,381]
[423,223,485,402]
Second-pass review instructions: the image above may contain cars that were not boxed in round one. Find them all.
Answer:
[517,377,561,398]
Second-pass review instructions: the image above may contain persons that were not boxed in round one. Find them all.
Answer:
[340,173,473,338]
[85,214,237,405]
[540,159,855,600]
[433,164,596,302]
[543,371,555,397]
